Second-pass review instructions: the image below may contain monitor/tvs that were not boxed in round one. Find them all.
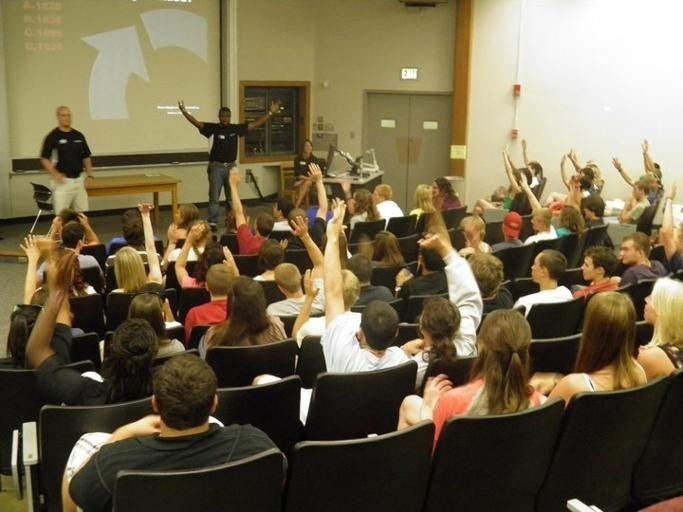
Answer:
[349,155,363,176]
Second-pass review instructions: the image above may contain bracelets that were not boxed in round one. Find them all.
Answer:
[182,110,186,114]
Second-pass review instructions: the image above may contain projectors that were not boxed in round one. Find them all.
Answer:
[399,0,448,7]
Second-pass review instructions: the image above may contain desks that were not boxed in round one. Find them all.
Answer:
[334,168,384,199]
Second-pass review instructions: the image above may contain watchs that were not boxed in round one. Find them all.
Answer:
[266,110,274,117]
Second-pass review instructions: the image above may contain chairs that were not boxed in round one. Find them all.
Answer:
[0,174,683,512]
[272,161,312,209]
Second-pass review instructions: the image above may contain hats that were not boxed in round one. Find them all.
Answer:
[502,212,522,238]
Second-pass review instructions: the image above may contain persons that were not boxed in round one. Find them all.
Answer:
[175,94,279,232]
[38,106,95,250]
[0,139,682,512]
